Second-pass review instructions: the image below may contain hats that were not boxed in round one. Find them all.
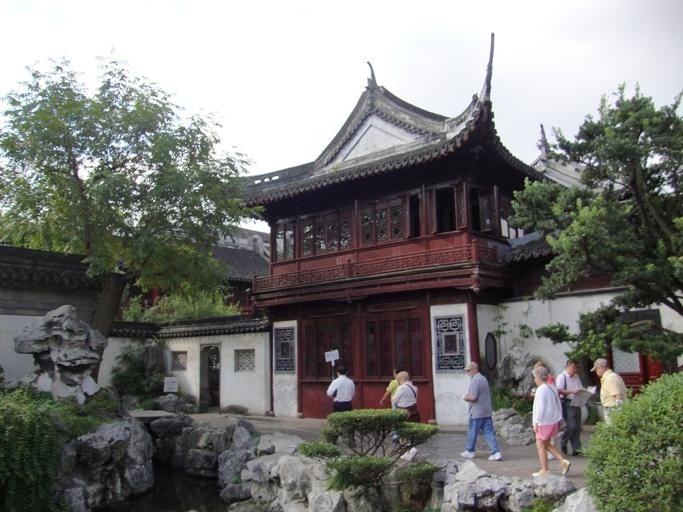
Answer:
[464,361,477,371]
[591,358,608,372]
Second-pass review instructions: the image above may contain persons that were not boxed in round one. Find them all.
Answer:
[379,366,406,447]
[533,359,559,460]
[459,362,503,461]
[531,366,571,478]
[591,358,630,424]
[326,365,357,450]
[391,371,419,462]
[555,359,596,457]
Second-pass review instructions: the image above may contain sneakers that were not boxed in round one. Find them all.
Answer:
[560,459,571,475]
[532,469,550,477]
[562,448,584,456]
[488,452,501,461]
[460,450,475,458]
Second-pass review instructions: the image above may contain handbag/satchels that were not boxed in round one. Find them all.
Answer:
[561,401,567,419]
